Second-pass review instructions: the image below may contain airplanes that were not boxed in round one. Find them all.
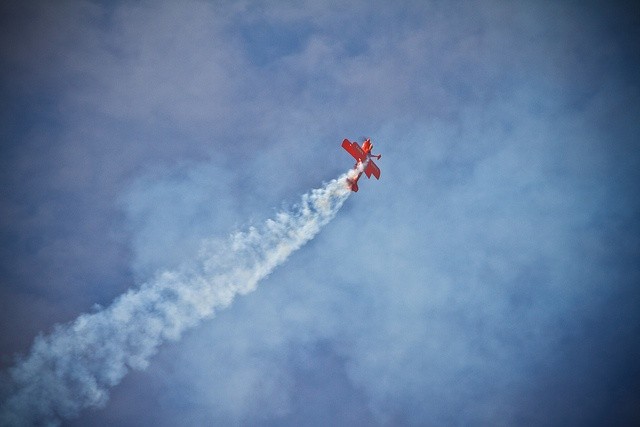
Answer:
[341,137,381,192]
